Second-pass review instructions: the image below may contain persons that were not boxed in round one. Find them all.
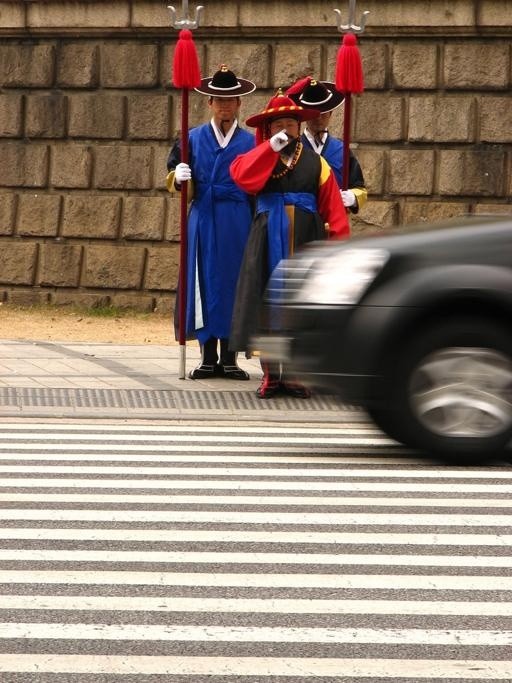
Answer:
[230,117,352,402]
[166,94,255,381]
[300,111,367,215]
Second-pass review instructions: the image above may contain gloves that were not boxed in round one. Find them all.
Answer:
[174,164,191,185]
[269,130,290,152]
[339,188,355,207]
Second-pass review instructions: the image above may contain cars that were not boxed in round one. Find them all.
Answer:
[246,215,511,461]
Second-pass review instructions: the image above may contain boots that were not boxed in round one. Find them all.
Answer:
[189,338,250,380]
[256,359,311,398]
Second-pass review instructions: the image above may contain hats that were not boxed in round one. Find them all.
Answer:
[192,66,256,98]
[245,78,345,129]
